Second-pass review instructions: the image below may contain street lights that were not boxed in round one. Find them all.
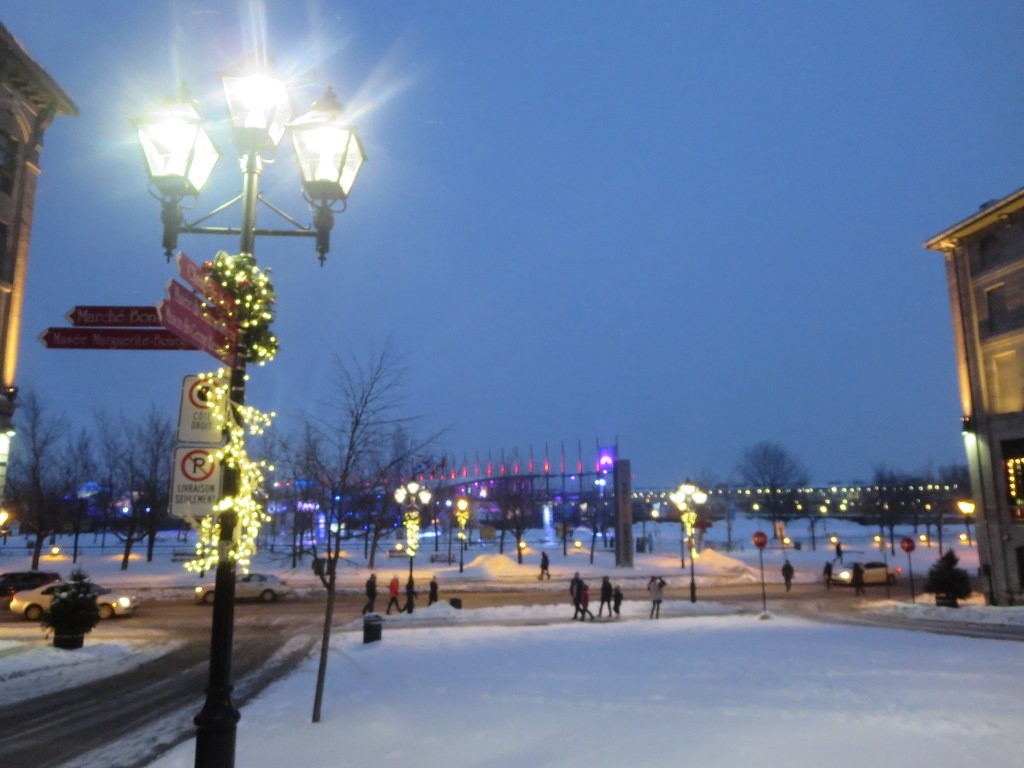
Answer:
[455,498,469,571]
[958,497,978,548]
[669,476,708,603]
[132,37,363,767]
[392,474,434,617]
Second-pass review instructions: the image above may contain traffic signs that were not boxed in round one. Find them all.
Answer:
[35,250,242,365]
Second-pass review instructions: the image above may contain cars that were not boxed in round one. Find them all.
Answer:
[0,569,62,609]
[9,579,146,620]
[830,560,903,585]
[191,571,289,602]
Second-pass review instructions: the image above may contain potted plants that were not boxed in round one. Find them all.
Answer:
[922,549,973,607]
[41,565,112,649]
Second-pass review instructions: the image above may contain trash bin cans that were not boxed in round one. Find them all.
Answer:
[936,590,958,607]
[637,537,645,552]
[450,597,462,609]
[54,594,84,648]
[364,615,382,643]
[314,558,325,575]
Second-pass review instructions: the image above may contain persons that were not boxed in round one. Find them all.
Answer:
[647,576,667,619]
[833,543,844,564]
[403,577,418,613]
[782,559,794,592]
[851,563,866,596]
[613,588,623,618]
[579,585,595,621]
[597,576,613,618]
[823,561,832,588]
[362,573,377,615]
[539,552,552,580]
[386,576,402,614]
[570,572,583,620]
[428,576,438,605]
